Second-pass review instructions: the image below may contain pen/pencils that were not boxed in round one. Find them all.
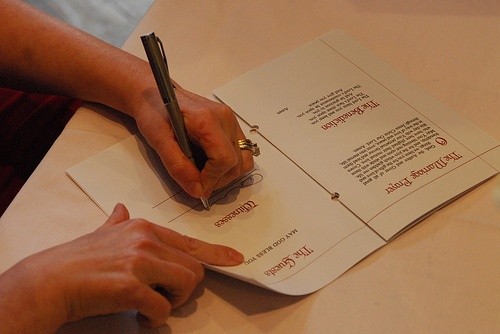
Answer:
[141,30,213,211]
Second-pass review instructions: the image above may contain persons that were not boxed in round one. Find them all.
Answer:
[0,1,256,334]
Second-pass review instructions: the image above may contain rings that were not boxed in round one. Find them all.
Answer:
[238,138,259,156]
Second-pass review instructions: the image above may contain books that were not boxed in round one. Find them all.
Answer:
[63,29,499,297]
